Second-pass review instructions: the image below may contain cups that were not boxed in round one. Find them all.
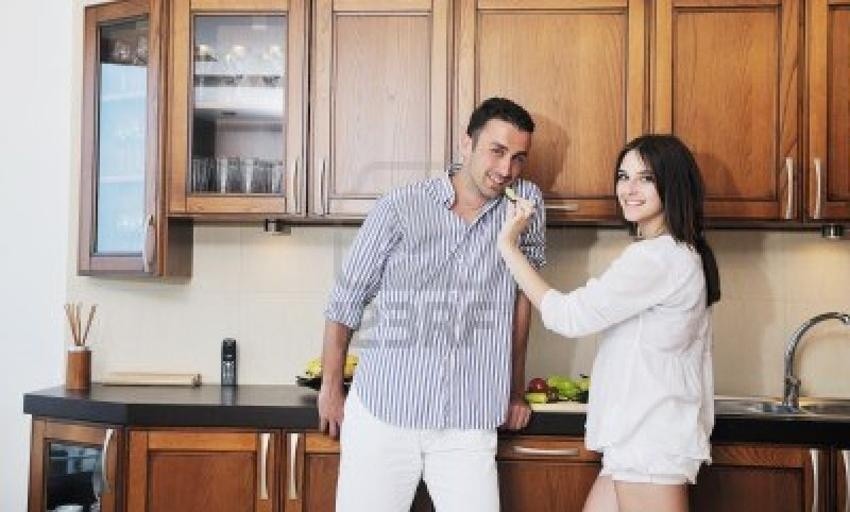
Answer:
[191,156,286,193]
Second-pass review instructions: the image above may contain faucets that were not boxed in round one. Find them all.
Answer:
[784,312,850,378]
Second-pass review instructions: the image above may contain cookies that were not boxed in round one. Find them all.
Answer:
[505,186,517,203]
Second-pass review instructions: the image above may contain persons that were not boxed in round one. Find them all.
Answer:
[316,94,546,512]
[495,132,716,512]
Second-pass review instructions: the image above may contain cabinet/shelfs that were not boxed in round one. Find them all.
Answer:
[128,427,431,512]
[28,418,125,512]
[648,0,850,230]
[78,1,193,278]
[686,447,850,512]
[167,0,453,226]
[453,0,649,228]
[430,436,602,511]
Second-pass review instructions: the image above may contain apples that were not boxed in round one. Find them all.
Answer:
[530,378,547,393]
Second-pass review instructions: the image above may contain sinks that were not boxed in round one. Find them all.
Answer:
[714,397,776,415]
[800,403,850,419]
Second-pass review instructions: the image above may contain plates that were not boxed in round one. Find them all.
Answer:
[295,375,356,397]
